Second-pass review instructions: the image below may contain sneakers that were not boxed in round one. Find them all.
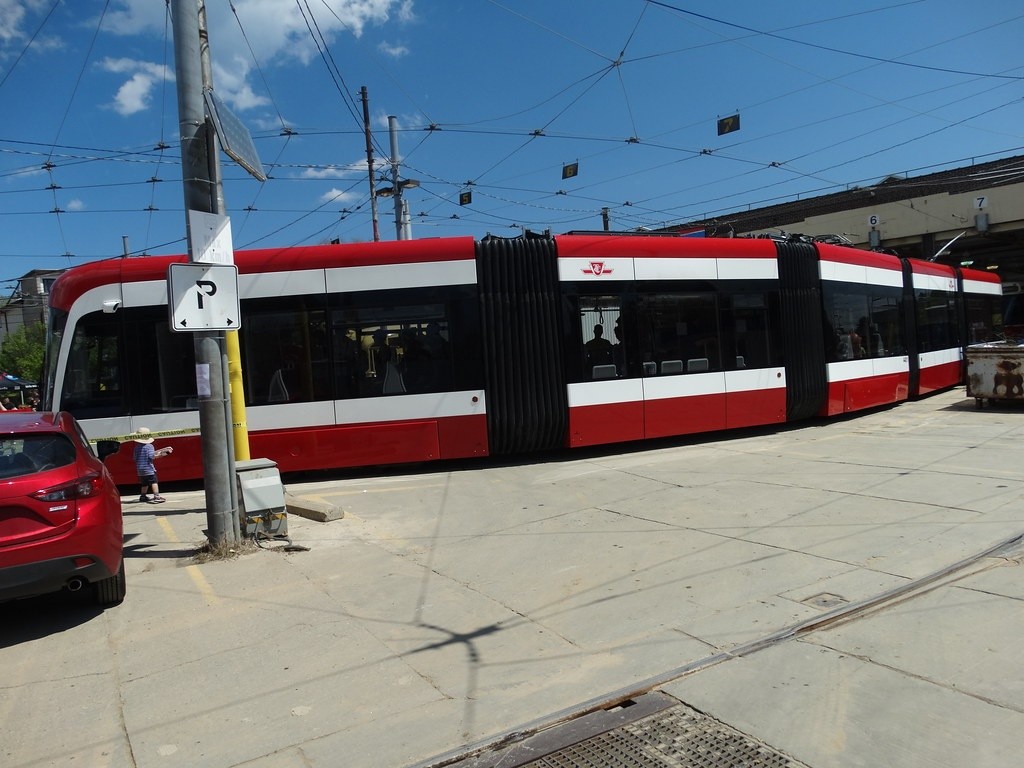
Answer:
[140,494,154,502]
[154,496,166,503]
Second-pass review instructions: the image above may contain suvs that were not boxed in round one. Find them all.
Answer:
[0,409,128,609]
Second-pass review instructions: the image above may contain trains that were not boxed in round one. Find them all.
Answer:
[50,230,1023,485]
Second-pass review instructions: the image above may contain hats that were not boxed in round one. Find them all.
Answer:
[130,427,155,444]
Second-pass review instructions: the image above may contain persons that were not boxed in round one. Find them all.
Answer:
[0,277,1014,417]
[128,428,173,503]
